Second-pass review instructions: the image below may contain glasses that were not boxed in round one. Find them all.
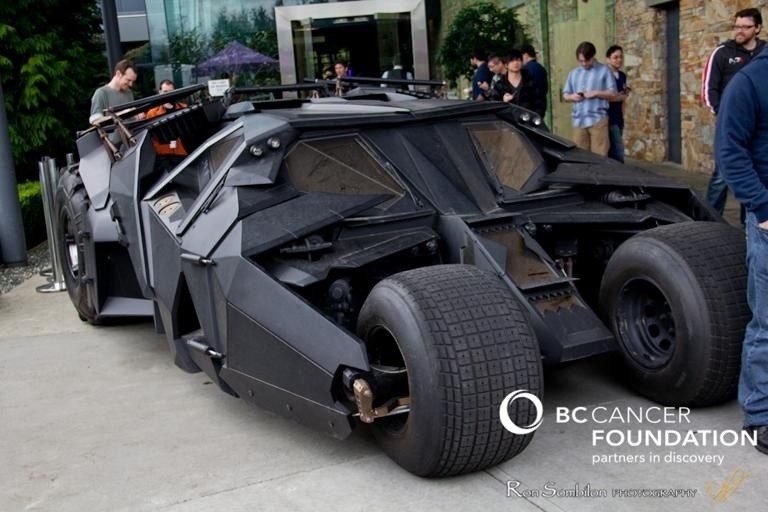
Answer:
[731,24,756,30]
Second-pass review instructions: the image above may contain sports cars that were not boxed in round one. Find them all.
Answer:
[54,79,752,480]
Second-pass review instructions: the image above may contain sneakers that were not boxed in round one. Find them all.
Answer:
[744,423,768,454]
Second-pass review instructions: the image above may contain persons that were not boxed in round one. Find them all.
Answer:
[700,7,765,226]
[606,45,632,163]
[88,57,146,128]
[713,38,767,462]
[145,79,189,159]
[312,41,550,124]
[561,41,618,156]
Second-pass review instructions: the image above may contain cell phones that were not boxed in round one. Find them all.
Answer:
[577,92,584,98]
